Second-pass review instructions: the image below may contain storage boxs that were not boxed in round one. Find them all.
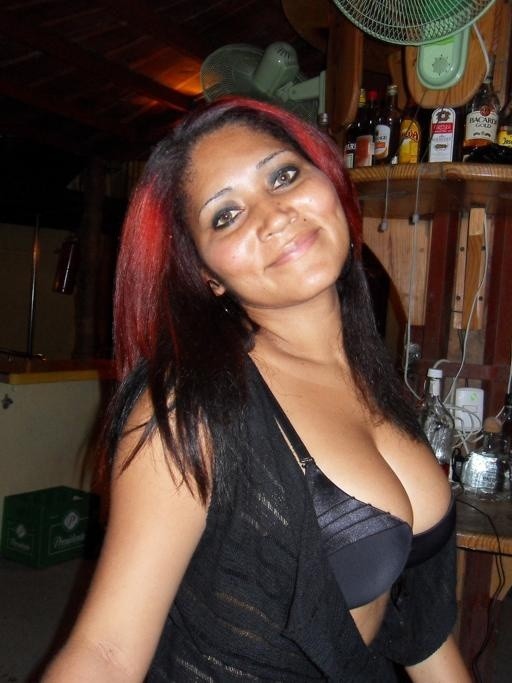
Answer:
[0,485,101,569]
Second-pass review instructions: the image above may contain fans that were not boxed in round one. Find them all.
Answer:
[200,40,328,132]
[333,0,498,90]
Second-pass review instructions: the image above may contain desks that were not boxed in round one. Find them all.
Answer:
[452,486,511,649]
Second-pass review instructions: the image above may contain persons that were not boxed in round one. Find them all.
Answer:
[41,95,475,682]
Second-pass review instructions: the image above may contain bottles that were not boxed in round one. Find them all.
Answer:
[458,415,502,498]
[344,87,380,167]
[313,112,337,144]
[462,49,500,165]
[492,97,511,164]
[373,83,401,165]
[415,368,454,481]
[496,393,512,503]
[399,113,421,163]
